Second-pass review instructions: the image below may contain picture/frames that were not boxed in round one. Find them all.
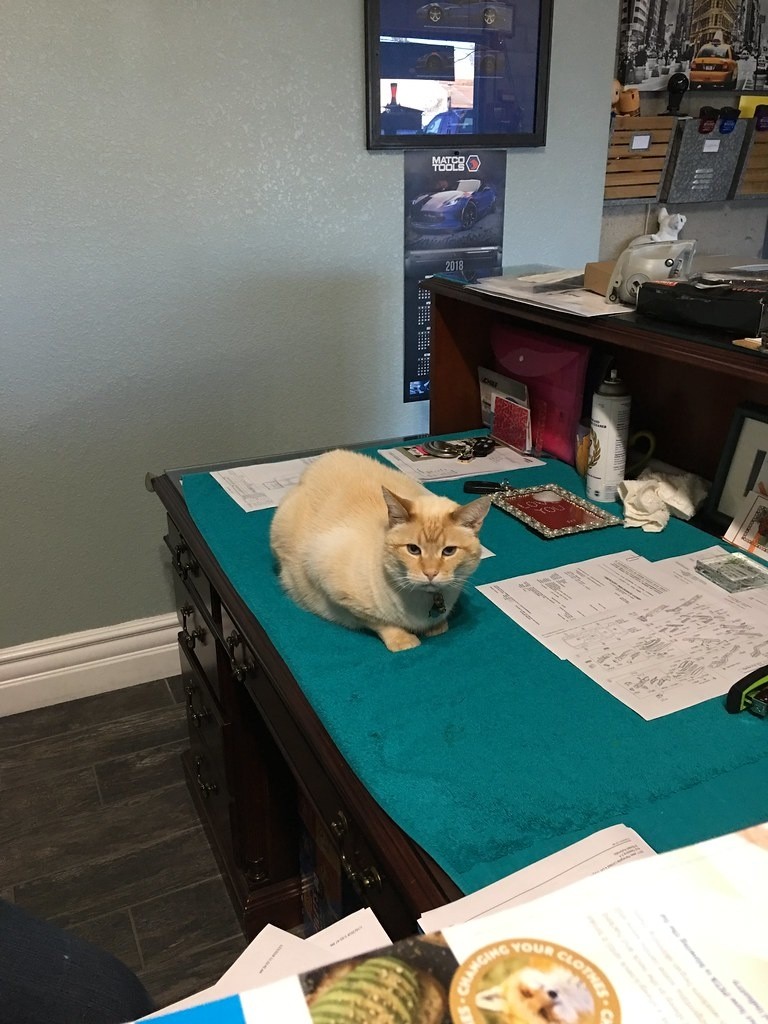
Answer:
[364,0,554,150]
[705,400,768,529]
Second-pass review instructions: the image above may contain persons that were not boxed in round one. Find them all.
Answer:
[635,37,761,78]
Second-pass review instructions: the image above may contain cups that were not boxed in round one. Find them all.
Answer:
[574,414,655,481]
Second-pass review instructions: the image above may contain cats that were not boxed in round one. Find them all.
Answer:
[269,449,495,653]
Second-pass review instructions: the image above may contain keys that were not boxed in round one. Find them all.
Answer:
[464,481,501,493]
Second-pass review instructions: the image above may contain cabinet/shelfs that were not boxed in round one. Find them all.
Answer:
[151,263,767,945]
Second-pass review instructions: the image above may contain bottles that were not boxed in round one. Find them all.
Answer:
[586,367,631,502]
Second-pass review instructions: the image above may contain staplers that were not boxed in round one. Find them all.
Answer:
[726,665,768,719]
